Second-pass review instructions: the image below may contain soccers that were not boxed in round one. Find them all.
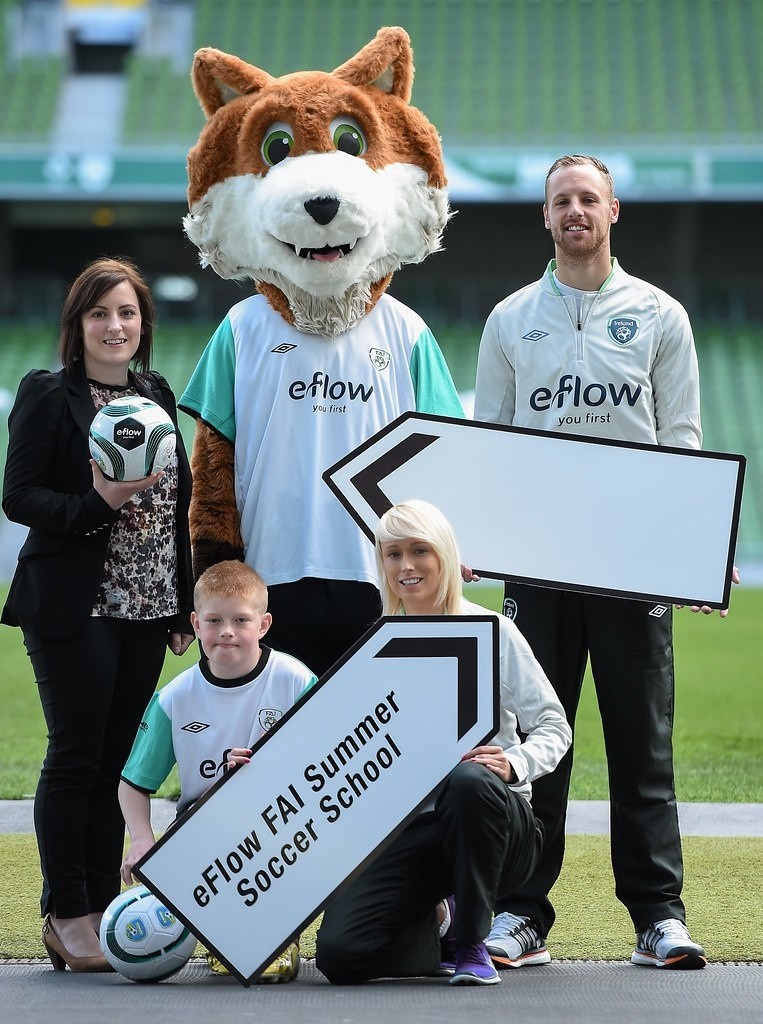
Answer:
[99,887,198,981]
[90,392,178,480]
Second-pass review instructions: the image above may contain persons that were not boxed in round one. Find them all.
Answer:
[118,560,318,981]
[317,498,571,986]
[479,155,740,970]
[0,254,196,970]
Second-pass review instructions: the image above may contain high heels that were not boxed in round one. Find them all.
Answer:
[40,914,116,972]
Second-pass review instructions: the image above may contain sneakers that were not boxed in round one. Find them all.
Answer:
[449,941,501,986]
[259,939,300,983]
[483,911,551,967]
[203,950,231,976]
[630,918,706,969]
[439,894,456,976]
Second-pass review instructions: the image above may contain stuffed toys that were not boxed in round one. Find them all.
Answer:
[176,24,479,676]
